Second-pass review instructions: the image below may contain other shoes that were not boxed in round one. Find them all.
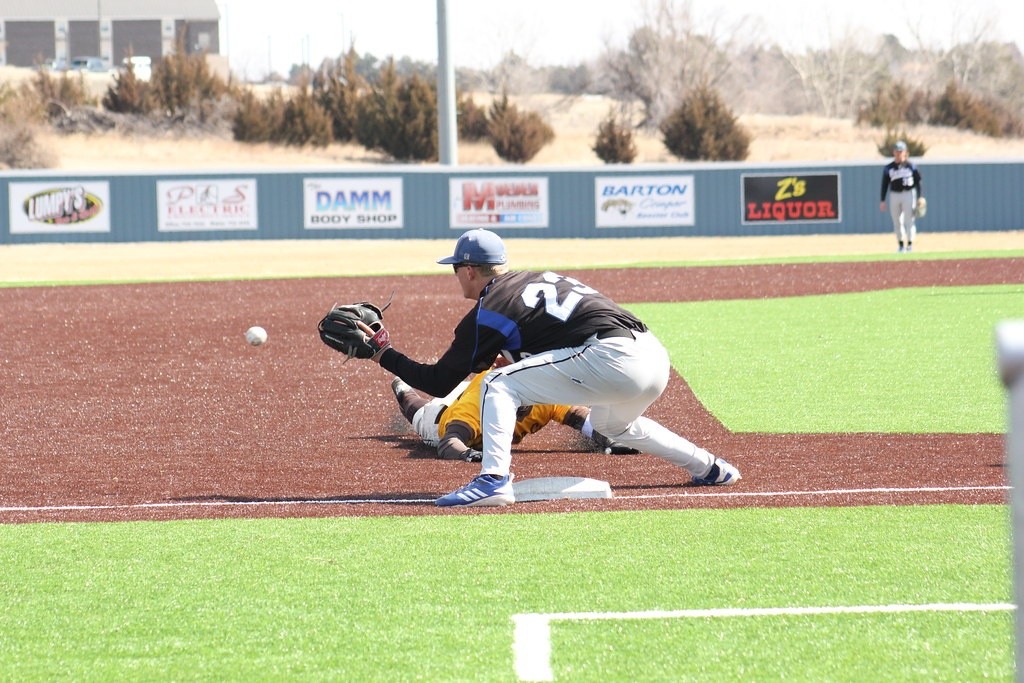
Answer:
[898,244,913,251]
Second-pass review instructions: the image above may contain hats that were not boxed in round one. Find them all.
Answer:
[436,228,508,265]
[894,141,908,150]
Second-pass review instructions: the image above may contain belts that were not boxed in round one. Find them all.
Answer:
[572,328,634,348]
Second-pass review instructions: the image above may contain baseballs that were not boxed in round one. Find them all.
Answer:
[246,326,266,344]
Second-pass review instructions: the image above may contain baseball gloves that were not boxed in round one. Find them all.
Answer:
[318,290,394,364]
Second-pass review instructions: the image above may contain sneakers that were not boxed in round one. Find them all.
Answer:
[392,375,413,394]
[435,473,515,507]
[691,457,740,486]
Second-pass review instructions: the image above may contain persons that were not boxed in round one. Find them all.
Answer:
[317,228,742,509]
[391,354,644,463]
[880,140,926,253]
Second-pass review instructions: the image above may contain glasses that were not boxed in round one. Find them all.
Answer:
[452,263,482,273]
